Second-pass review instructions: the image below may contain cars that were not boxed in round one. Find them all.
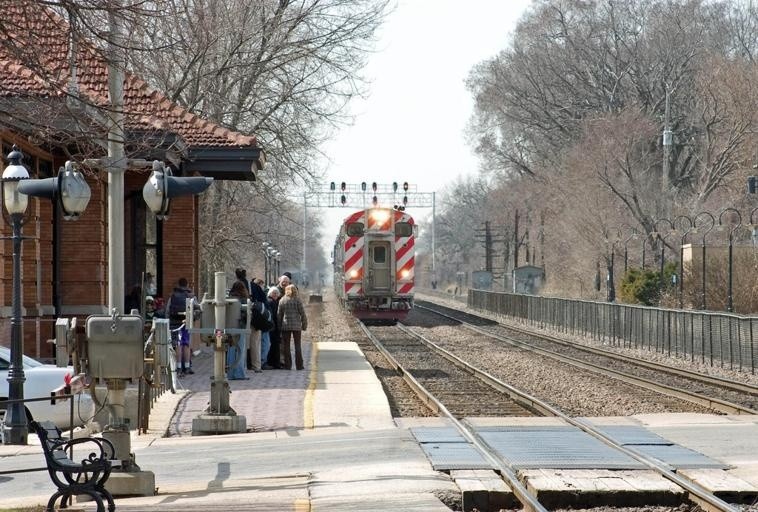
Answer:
[0,343,94,444]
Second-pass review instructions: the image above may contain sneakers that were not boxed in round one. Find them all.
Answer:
[177,368,194,374]
[252,364,304,373]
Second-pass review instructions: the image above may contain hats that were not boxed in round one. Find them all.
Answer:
[145,273,155,278]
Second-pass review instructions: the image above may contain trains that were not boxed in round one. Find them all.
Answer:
[330,208,419,330]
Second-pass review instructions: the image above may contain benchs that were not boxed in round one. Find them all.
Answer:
[28,420,122,512]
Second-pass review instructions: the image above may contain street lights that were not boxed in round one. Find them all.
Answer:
[0,141,32,449]
[262,240,281,287]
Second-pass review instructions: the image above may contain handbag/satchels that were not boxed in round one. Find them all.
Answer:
[251,302,275,332]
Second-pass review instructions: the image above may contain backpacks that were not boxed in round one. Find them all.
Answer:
[170,288,189,319]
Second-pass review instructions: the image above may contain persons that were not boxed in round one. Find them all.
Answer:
[145,266,308,380]
